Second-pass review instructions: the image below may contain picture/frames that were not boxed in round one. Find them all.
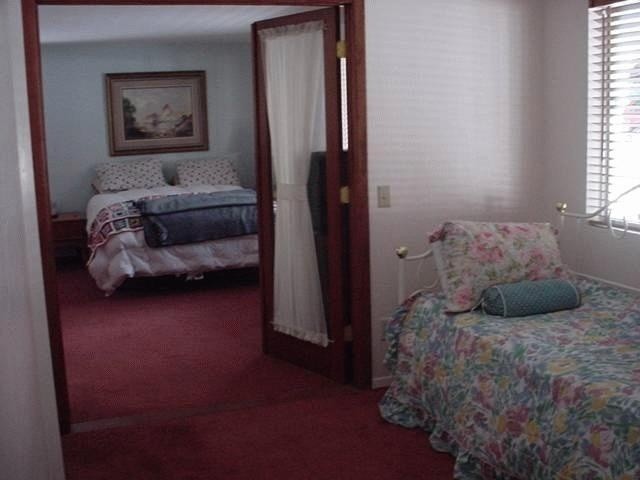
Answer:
[103,69,210,156]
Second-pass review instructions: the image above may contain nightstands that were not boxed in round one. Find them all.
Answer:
[51,212,88,267]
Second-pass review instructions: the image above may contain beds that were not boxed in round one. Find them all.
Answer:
[89,158,259,287]
[396,187,639,480]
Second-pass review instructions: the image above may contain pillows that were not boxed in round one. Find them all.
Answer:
[91,160,241,192]
[429,217,581,315]
